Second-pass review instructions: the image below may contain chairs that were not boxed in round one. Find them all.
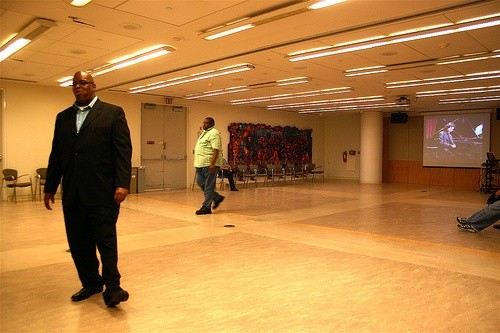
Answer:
[2,169,34,204]
[193,161,324,191]
[34,168,61,202]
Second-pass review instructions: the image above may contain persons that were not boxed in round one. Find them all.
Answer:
[438,123,456,148]
[456,200,500,233]
[43,71,132,308]
[218,153,239,191]
[194,117,225,215]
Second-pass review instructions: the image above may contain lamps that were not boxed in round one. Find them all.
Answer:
[0,0,345,93]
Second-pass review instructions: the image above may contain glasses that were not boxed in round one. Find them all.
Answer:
[69,80,93,87]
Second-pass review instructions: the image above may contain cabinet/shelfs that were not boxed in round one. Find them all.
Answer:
[480,159,500,194]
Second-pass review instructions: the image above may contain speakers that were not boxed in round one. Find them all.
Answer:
[496,108,500,120]
[390,113,408,123]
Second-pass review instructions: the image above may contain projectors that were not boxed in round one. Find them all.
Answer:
[395,99,409,104]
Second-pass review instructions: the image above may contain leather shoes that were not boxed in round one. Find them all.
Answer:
[70,286,104,301]
[103,286,129,306]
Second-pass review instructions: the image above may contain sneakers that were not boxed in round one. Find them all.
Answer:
[456,215,477,233]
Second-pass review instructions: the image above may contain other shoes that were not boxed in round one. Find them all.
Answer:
[195,207,212,214]
[231,188,240,192]
[213,195,225,209]
